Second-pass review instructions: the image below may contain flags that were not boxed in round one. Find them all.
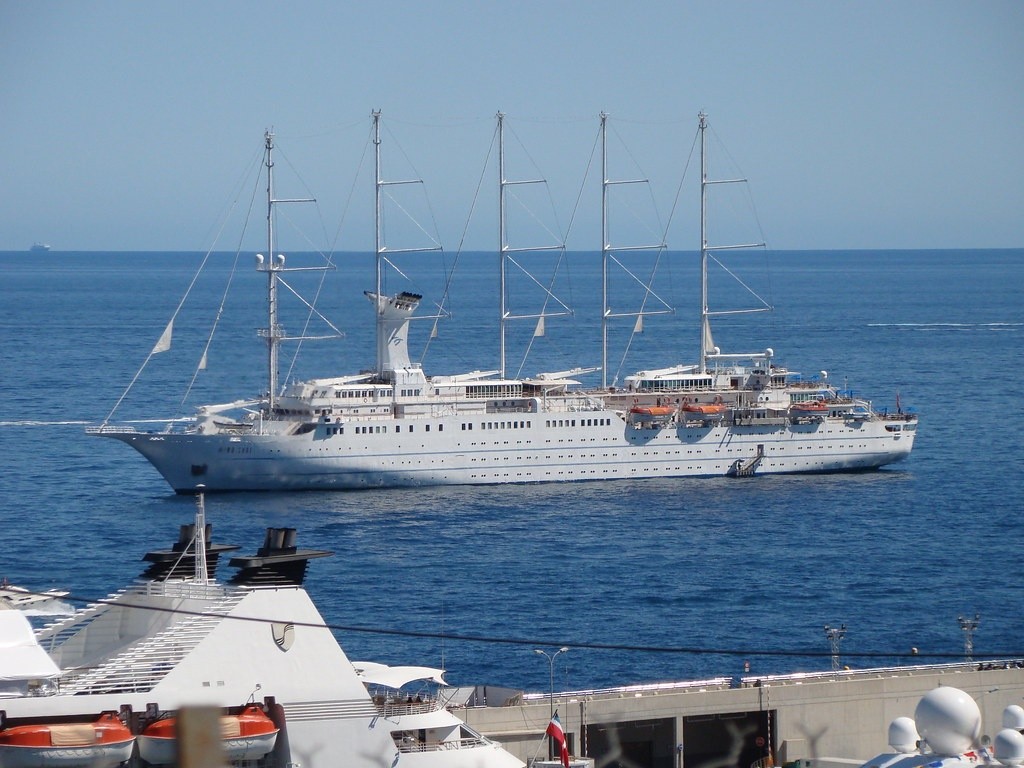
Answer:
[546,709,569,768]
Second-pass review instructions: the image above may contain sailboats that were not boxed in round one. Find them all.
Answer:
[83,107,918,494]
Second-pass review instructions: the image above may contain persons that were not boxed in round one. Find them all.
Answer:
[406,696,423,708]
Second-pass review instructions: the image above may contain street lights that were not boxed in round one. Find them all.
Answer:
[534,648,569,761]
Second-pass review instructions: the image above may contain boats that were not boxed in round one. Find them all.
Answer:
[629,407,675,422]
[681,403,727,420]
[136,708,280,768]
[0,711,136,768]
[0,483,529,768]
[790,401,830,416]
